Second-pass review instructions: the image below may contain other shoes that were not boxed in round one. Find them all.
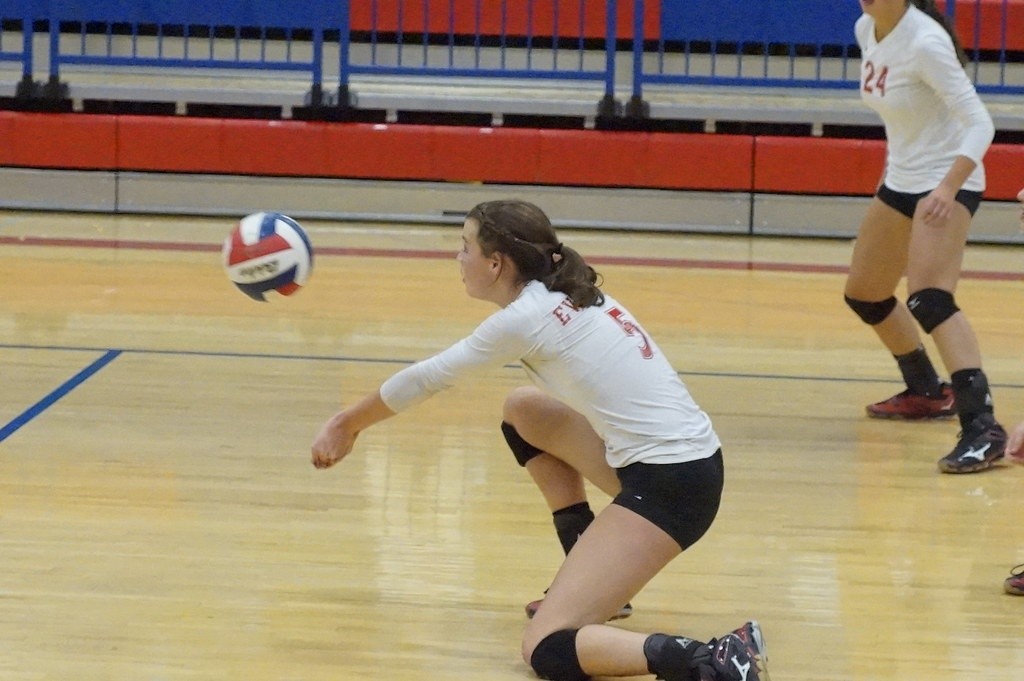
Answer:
[1004,569,1024,596]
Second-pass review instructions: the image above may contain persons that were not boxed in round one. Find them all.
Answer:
[843,1,1010,474]
[310,199,774,681]
[1003,185,1024,596]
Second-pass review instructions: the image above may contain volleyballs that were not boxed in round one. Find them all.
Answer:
[220,211,316,306]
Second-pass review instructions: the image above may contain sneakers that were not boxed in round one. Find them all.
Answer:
[525,584,633,620]
[937,418,1011,474]
[864,382,960,422]
[708,619,770,680]
